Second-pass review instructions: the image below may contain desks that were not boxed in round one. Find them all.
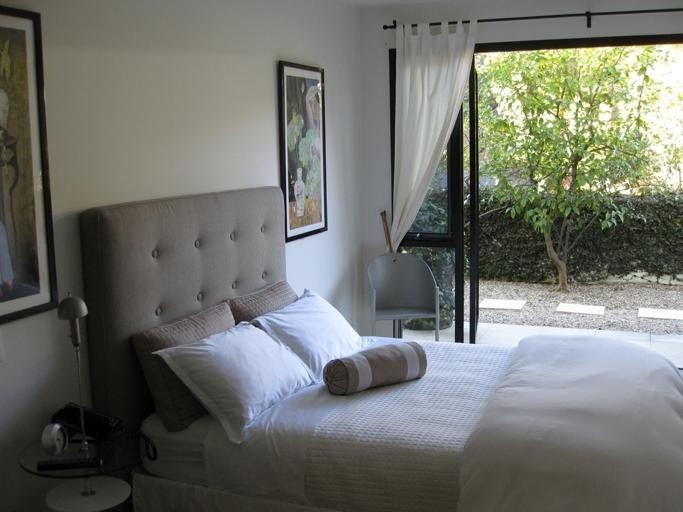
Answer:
[19,430,150,510]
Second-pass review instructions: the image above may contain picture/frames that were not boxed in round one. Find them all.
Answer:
[0,5,59,326]
[279,60,328,241]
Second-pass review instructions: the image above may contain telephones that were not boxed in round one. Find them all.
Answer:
[51,402,127,445]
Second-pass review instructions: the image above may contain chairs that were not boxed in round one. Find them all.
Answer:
[367,252,440,349]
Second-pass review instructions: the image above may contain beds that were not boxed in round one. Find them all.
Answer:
[80,181,683,512]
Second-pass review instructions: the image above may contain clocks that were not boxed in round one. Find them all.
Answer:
[41,423,69,457]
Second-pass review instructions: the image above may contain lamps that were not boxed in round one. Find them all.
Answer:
[35,288,112,471]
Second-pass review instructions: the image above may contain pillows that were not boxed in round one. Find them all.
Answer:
[141,301,235,433]
[252,287,364,385]
[152,321,314,445]
[323,342,426,394]
[226,280,298,323]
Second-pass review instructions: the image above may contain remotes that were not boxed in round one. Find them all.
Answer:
[37,458,103,472]
